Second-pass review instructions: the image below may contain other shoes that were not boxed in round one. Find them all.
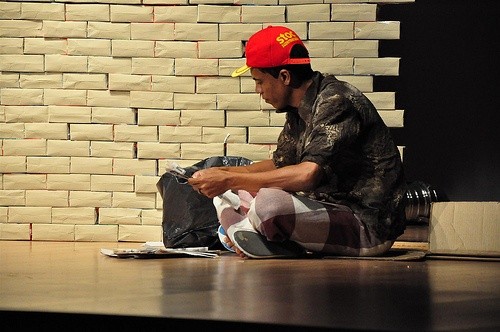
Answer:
[234,230,307,258]
[218,225,236,252]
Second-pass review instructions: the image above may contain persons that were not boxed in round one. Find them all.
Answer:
[188,25,407,259]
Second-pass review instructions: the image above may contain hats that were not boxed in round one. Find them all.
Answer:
[231,25,310,77]
